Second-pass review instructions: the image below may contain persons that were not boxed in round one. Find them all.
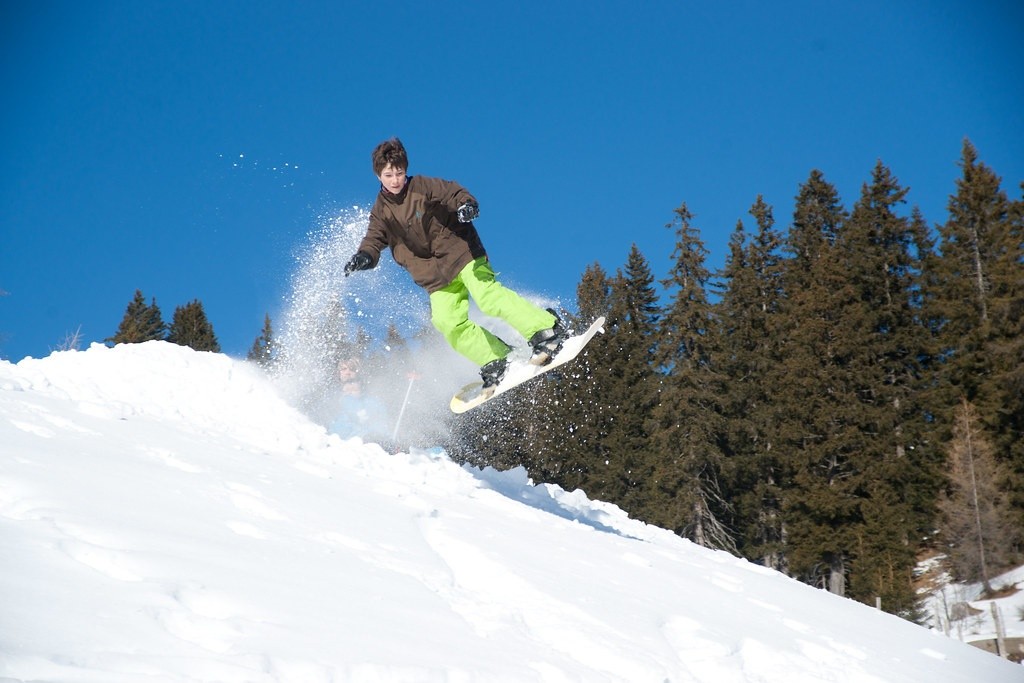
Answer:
[343,139,565,388]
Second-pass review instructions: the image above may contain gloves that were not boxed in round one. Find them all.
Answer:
[344,251,374,276]
[457,204,479,223]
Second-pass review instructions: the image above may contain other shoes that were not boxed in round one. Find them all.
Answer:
[528,308,570,366]
[479,344,515,389]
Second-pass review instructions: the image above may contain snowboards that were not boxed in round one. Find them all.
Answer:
[447,316,610,415]
